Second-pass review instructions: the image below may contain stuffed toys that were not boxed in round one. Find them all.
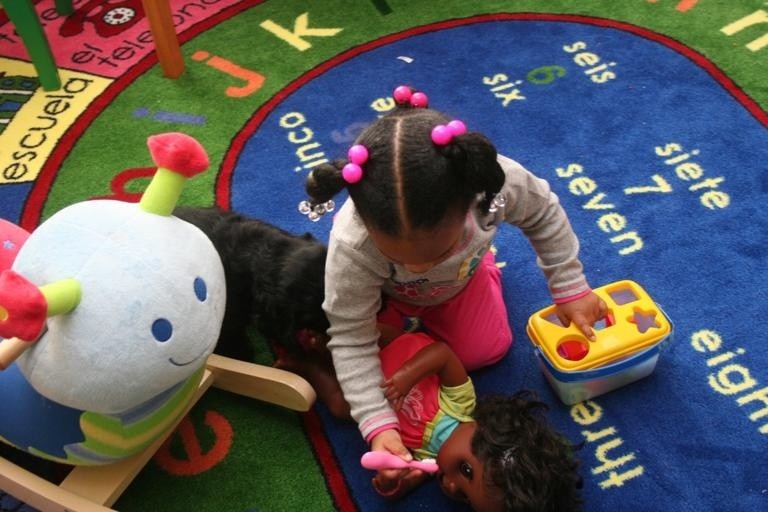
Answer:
[0,130,316,512]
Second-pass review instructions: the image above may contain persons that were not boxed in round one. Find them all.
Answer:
[270,323,587,512]
[303,83,608,486]
